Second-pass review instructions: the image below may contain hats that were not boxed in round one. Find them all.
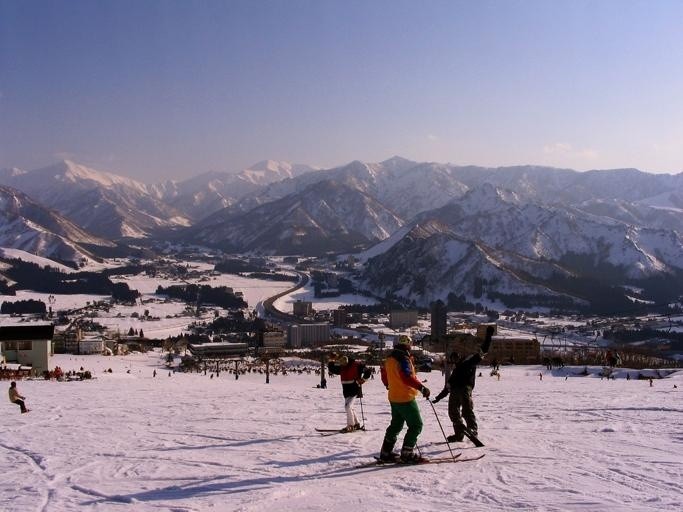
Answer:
[399,334,413,348]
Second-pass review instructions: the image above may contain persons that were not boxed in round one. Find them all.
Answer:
[9,381,29,413]
[433,336,478,441]
[540,373,542,380]
[379,335,430,464]
[328,352,371,433]
[153,370,156,377]
[627,373,630,380]
[321,379,327,389]
[650,378,653,387]
[565,373,569,380]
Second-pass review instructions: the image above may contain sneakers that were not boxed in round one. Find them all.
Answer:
[400,453,422,464]
[343,423,360,432]
[379,451,400,461]
[467,427,477,436]
[447,435,463,442]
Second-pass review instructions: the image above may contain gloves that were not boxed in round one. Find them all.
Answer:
[421,386,430,398]
[355,378,365,385]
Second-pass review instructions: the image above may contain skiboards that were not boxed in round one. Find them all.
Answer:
[356,453,486,468]
[314,426,379,436]
